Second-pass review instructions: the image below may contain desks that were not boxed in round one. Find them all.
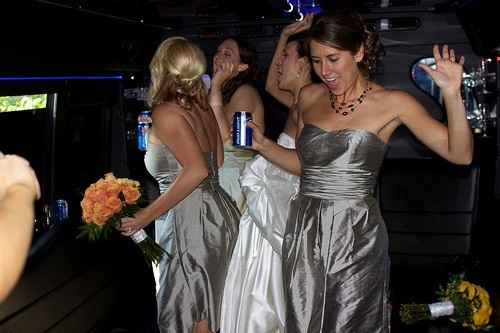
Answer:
[389,252,500,333]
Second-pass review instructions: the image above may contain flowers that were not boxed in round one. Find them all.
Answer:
[399,274,492,330]
[74,172,171,267]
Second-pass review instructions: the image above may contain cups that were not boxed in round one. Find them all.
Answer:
[124,87,149,100]
[34,204,56,233]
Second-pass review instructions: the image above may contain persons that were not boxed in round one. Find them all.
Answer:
[0,149,43,302]
[114,36,242,333]
[199,36,267,221]
[217,10,330,333]
[227,5,474,332]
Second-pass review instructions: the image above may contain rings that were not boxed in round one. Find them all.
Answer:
[451,59,455,61]
[128,228,132,233]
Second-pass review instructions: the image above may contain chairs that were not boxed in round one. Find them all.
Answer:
[375,157,481,254]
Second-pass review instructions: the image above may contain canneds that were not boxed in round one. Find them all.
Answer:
[55,199,68,221]
[232,111,253,148]
[137,111,152,150]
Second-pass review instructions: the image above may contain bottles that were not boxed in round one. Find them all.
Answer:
[125,112,140,150]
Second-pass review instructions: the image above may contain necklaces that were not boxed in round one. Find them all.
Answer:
[328,74,373,116]
